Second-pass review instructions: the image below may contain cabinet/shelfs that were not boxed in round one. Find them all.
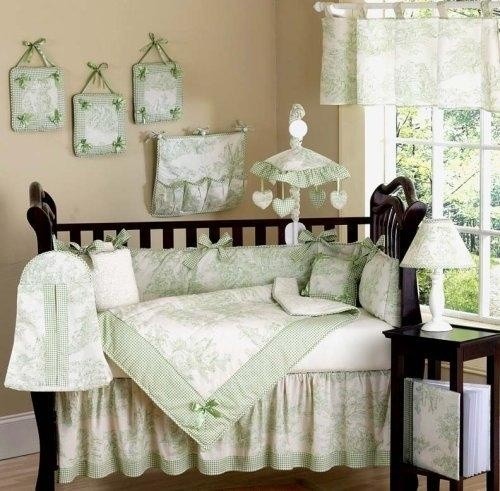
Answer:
[384,322,499,490]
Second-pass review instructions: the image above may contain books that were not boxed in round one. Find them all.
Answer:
[403,375,491,482]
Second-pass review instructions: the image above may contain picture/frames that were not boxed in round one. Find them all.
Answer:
[131,60,184,124]
[8,66,66,133]
[71,92,126,159]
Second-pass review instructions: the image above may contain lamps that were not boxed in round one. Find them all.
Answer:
[398,218,479,335]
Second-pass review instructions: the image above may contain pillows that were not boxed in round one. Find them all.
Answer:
[301,253,365,312]
[356,248,405,329]
[88,248,139,312]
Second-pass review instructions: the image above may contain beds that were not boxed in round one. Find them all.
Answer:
[21,173,424,490]
